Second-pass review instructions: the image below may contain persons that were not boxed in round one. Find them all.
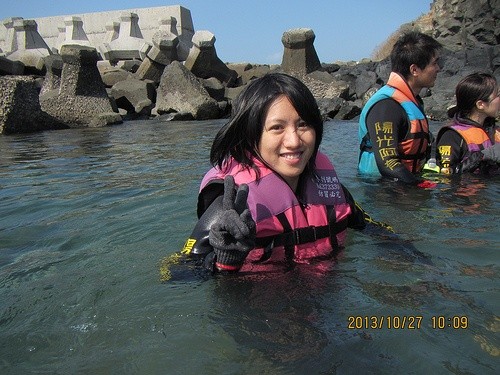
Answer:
[158,72,434,274]
[355,29,443,189]
[436,71,500,177]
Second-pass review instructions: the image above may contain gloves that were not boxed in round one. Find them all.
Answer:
[208,174,255,267]
